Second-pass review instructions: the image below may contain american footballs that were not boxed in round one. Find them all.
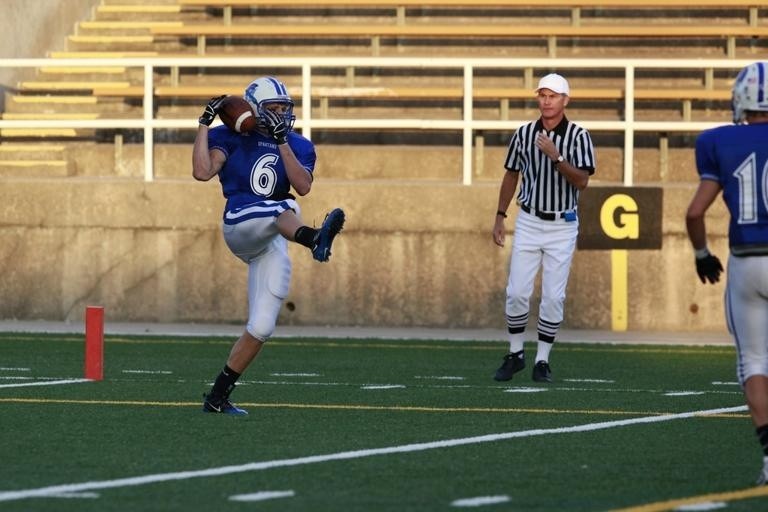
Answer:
[218,95,256,132]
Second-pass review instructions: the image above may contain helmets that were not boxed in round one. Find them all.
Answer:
[243,77,294,128]
[732,62,768,123]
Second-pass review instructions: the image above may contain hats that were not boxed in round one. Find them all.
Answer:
[533,73,570,95]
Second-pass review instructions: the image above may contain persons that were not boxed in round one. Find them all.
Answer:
[686,61,768,487]
[191,77,346,415]
[494,74,595,383]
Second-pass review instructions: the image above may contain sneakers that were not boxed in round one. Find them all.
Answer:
[311,208,345,262]
[532,360,552,382]
[495,354,526,381]
[203,393,248,415]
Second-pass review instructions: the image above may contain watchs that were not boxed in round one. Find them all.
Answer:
[553,155,564,164]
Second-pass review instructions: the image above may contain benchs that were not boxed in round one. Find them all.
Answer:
[90,1,768,179]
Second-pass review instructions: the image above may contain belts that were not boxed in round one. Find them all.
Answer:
[520,204,575,220]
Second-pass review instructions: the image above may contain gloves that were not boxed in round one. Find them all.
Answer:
[258,108,287,144]
[199,95,228,126]
[696,253,724,284]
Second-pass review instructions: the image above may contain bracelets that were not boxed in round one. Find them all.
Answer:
[496,210,507,218]
